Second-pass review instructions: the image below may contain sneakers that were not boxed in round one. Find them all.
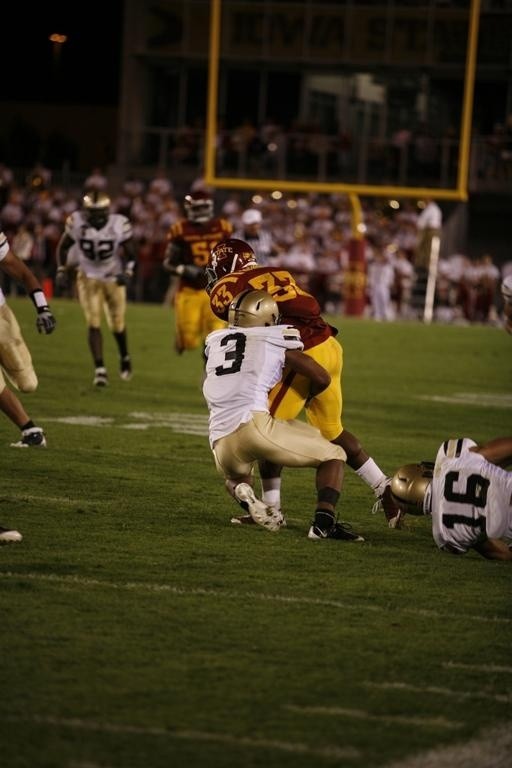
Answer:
[119,354,132,381]
[94,367,108,388]
[306,524,365,542]
[0,529,21,545]
[381,479,402,528]
[10,427,48,448]
[231,482,287,533]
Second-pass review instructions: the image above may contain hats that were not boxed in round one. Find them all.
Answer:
[241,210,262,225]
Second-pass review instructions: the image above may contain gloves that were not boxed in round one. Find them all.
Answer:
[55,268,73,288]
[176,264,202,281]
[113,272,134,285]
[36,311,57,335]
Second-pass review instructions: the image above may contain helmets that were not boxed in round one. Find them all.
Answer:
[229,290,280,328]
[83,192,111,216]
[182,190,214,224]
[390,461,434,517]
[205,238,257,295]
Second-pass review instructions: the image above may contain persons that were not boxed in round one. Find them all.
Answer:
[0,121,511,329]
[206,239,406,526]
[202,285,367,539]
[55,171,139,388]
[227,209,285,265]
[0,526,24,546]
[162,189,231,354]
[1,234,57,446]
[391,435,512,564]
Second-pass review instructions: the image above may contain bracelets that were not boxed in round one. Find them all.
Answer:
[175,263,185,275]
[28,289,51,313]
[127,260,136,268]
[57,266,66,272]
